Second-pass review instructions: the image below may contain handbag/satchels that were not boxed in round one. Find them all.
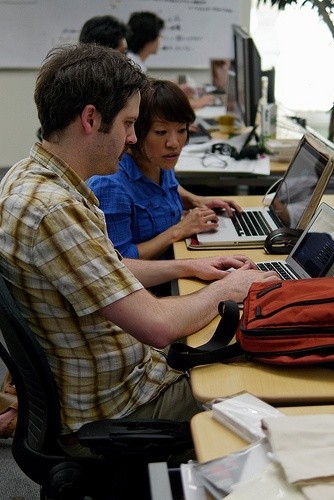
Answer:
[165,279,334,375]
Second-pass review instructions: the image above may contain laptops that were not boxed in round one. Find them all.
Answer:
[222,202,334,281]
[190,126,334,247]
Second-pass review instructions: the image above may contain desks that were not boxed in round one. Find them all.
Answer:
[174,119,292,195]
[172,195,334,500]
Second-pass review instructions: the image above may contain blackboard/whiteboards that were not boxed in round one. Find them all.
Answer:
[0,0,252,72]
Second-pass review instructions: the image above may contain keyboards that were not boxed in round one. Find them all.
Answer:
[186,123,211,144]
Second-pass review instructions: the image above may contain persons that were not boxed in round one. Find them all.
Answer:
[122,11,216,111]
[82,76,244,264]
[78,14,202,135]
[1,43,283,455]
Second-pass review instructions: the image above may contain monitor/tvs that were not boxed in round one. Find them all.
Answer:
[211,58,232,94]
[232,24,275,129]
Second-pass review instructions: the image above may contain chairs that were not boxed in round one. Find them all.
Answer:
[0,276,186,500]
